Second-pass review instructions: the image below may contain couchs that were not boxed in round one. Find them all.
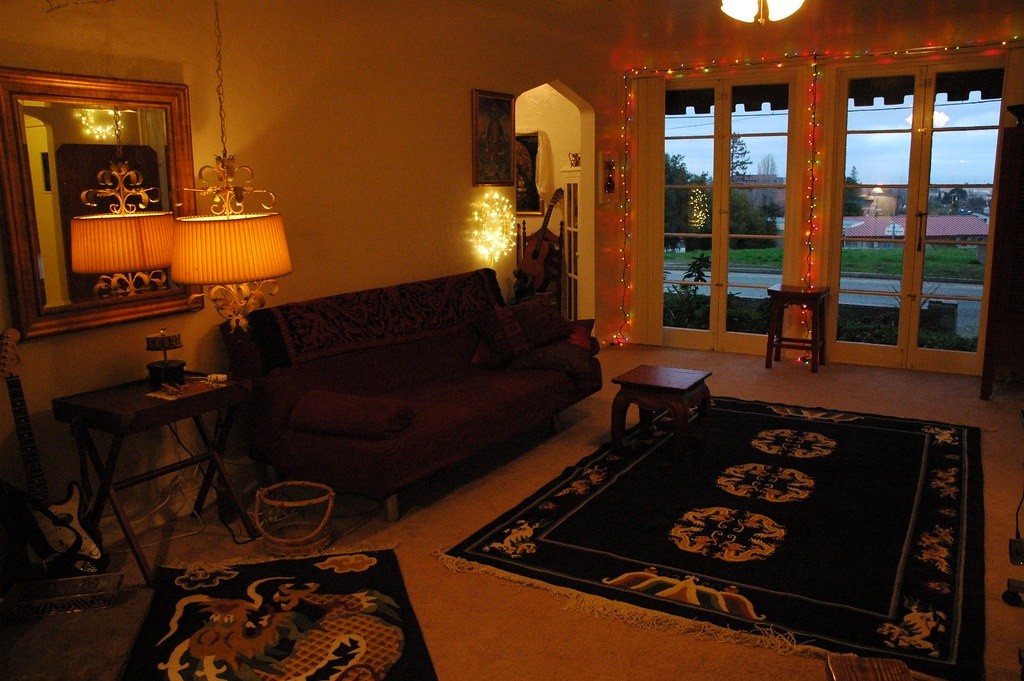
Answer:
[217,268,603,521]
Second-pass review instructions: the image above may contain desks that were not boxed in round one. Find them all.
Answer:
[765,284,831,372]
[611,365,713,458]
[52,371,260,586]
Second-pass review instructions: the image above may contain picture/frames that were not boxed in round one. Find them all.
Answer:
[516,132,545,217]
[471,89,516,187]
[598,151,620,203]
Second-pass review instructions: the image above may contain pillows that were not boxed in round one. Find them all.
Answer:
[291,390,416,439]
[589,336,600,355]
[477,297,573,364]
[513,318,596,373]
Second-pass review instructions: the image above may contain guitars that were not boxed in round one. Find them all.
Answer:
[511,184,569,296]
[1,327,104,563]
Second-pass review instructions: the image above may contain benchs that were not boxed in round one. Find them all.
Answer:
[826,655,914,681]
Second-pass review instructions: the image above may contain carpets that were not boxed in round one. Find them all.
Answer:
[114,537,440,681]
[428,396,999,681]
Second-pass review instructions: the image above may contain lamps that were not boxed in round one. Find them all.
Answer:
[720,0,807,25]
[145,327,186,388]
[169,1,296,334]
[71,106,174,298]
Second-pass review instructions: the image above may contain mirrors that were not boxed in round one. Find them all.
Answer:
[0,66,204,341]
